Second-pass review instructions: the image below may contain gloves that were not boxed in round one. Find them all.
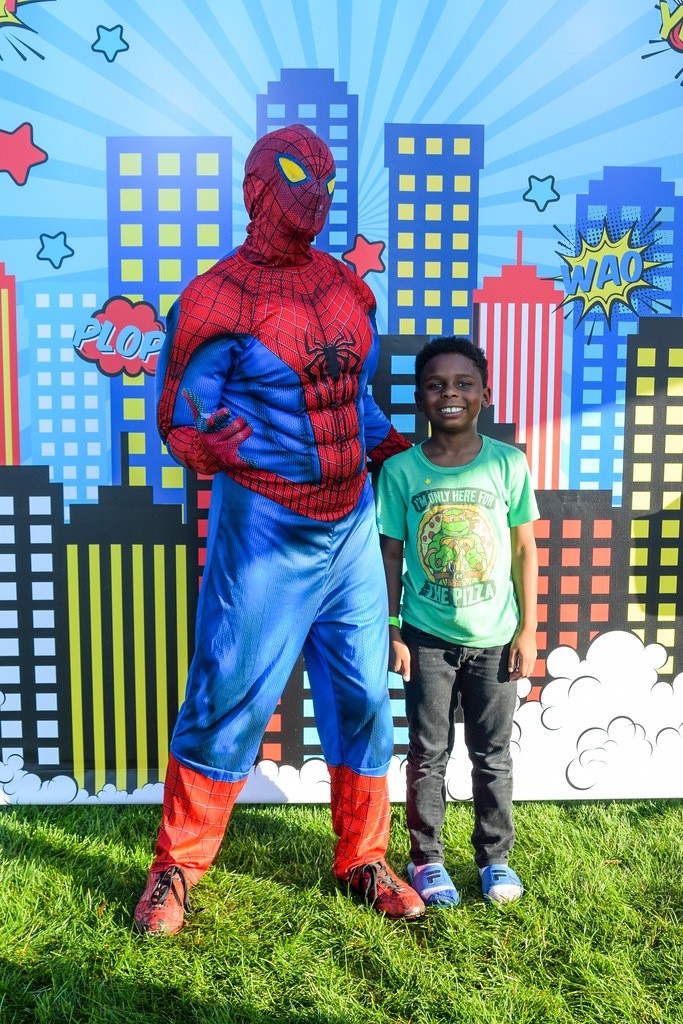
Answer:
[182,387,253,471]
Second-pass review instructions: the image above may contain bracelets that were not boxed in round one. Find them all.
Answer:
[388,617,401,627]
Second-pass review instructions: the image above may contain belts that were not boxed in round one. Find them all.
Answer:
[402,621,434,637]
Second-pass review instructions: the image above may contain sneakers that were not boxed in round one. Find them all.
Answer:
[134,865,205,937]
[337,860,425,920]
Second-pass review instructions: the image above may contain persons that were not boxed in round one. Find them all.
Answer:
[375,338,541,907]
[126,124,427,936]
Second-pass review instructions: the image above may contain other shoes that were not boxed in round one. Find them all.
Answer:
[478,864,521,904]
[407,862,459,907]
